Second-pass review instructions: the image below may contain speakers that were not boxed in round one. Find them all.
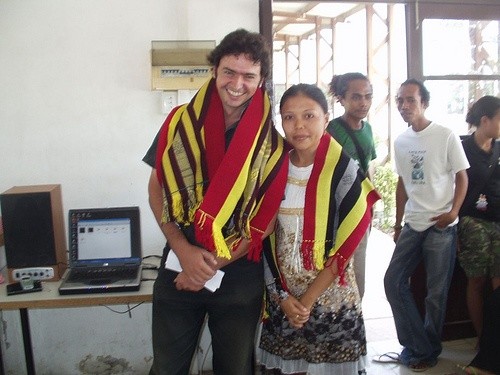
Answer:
[0,184,69,285]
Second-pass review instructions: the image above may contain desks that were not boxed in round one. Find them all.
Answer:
[0,261,158,375]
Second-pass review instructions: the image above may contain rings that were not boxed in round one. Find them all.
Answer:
[296,314,299,318]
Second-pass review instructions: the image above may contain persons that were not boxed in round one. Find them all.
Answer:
[141,29,286,375]
[459,96,500,329]
[326,73,377,299]
[384,80,470,364]
[256,83,381,375]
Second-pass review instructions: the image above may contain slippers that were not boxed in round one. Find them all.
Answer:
[373,352,404,362]
[456,364,479,375]
[408,359,426,371]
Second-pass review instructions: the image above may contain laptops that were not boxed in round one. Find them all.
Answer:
[58,207,144,296]
